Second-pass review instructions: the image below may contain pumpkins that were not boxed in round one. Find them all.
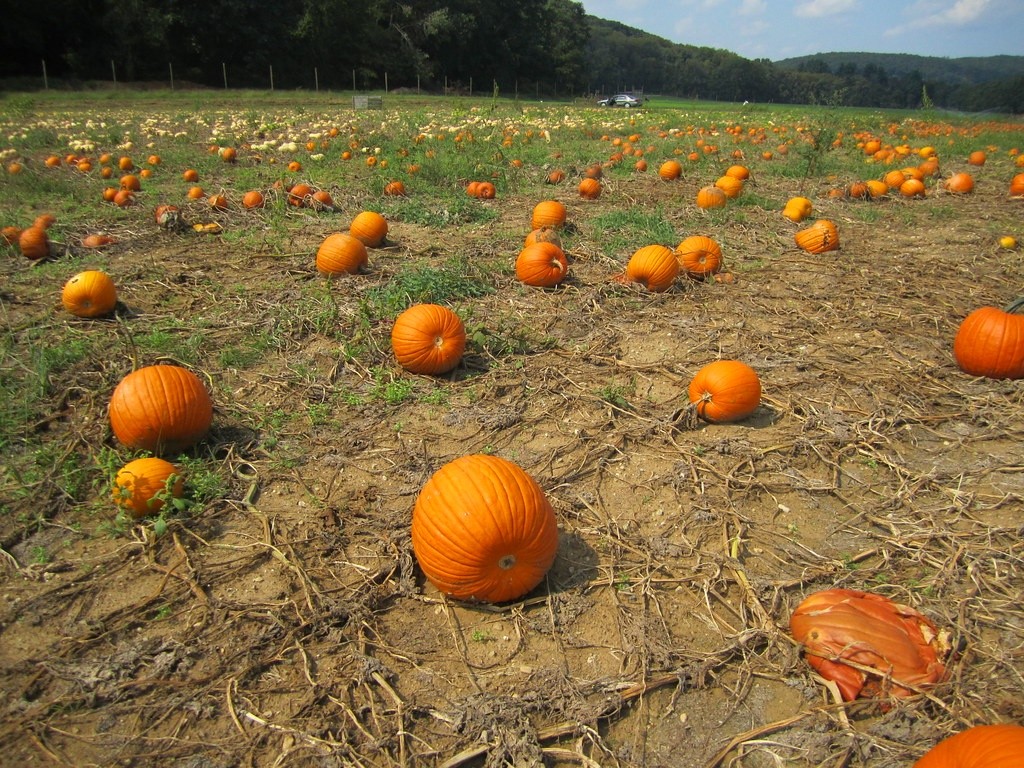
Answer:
[0,108,446,259]
[790,589,951,712]
[391,303,466,374]
[109,363,211,456]
[676,236,722,280]
[112,458,184,516]
[412,455,558,605]
[451,107,1024,255]
[624,244,681,293]
[63,270,116,318]
[909,723,1024,768]
[514,243,567,287]
[316,234,369,277]
[953,296,1024,380]
[688,359,760,423]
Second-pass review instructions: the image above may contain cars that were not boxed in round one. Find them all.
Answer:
[597,94,643,108]
